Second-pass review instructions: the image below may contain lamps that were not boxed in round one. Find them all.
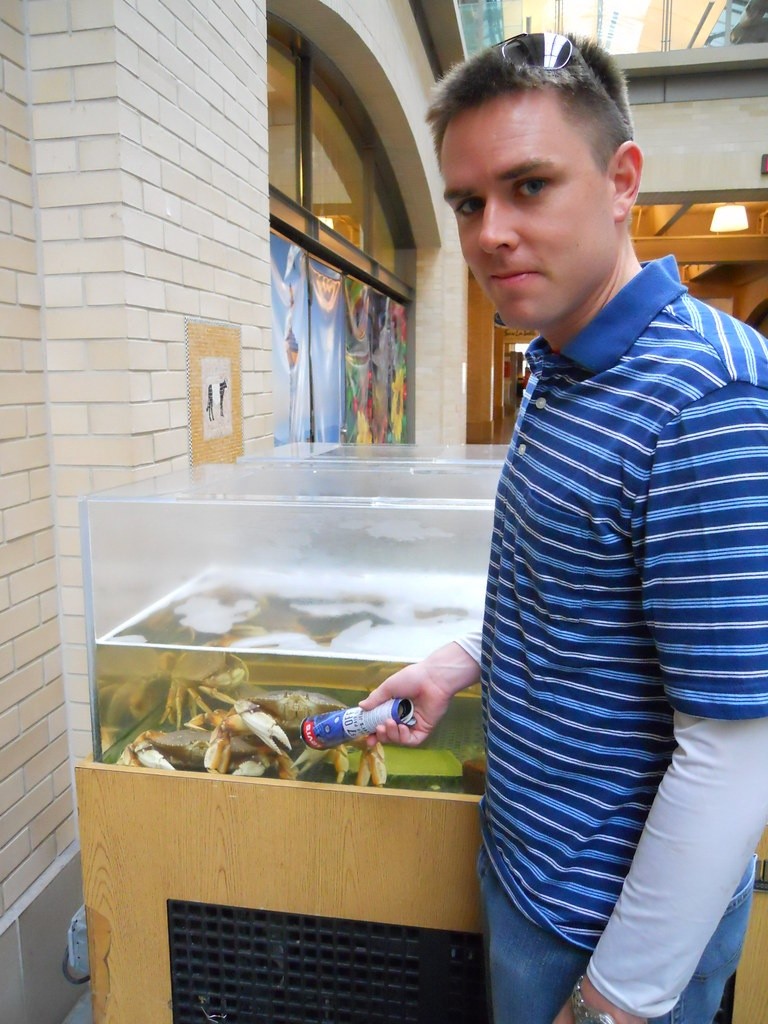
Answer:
[709,201,749,233]
[319,218,334,230]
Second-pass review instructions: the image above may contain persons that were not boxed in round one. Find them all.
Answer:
[358,34,768,1024]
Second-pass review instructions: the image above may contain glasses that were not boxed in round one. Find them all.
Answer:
[493,31,626,120]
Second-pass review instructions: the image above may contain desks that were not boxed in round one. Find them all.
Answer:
[74,755,768,1024]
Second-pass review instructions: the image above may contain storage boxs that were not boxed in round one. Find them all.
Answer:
[80,443,510,797]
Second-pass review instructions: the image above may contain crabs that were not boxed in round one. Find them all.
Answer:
[111,642,387,789]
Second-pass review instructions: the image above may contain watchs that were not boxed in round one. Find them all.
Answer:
[571,976,616,1024]
